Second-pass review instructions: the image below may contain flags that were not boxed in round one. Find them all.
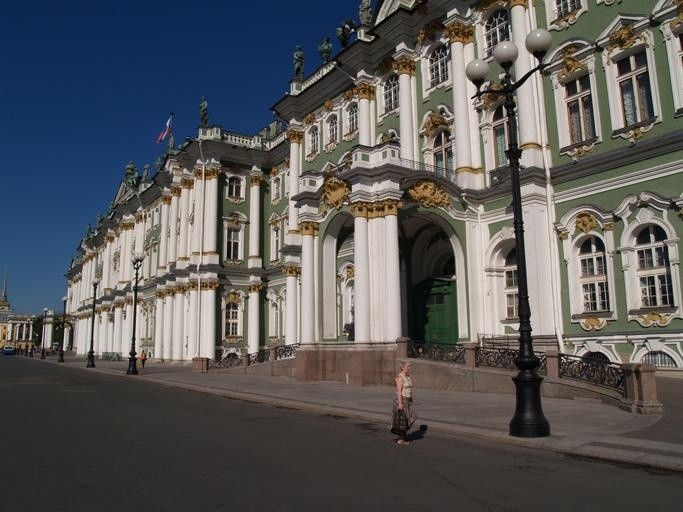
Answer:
[155,115,170,144]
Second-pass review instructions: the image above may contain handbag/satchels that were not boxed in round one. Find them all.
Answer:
[391,410,409,437]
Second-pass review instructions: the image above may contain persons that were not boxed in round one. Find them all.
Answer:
[391,359,417,444]
[139,349,146,368]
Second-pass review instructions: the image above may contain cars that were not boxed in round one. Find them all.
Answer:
[3,344,16,355]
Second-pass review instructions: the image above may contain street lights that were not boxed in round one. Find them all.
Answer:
[465,29,553,438]
[126,253,146,375]
[57,296,68,363]
[24,318,30,356]
[86,277,100,368]
[40,306,49,359]
